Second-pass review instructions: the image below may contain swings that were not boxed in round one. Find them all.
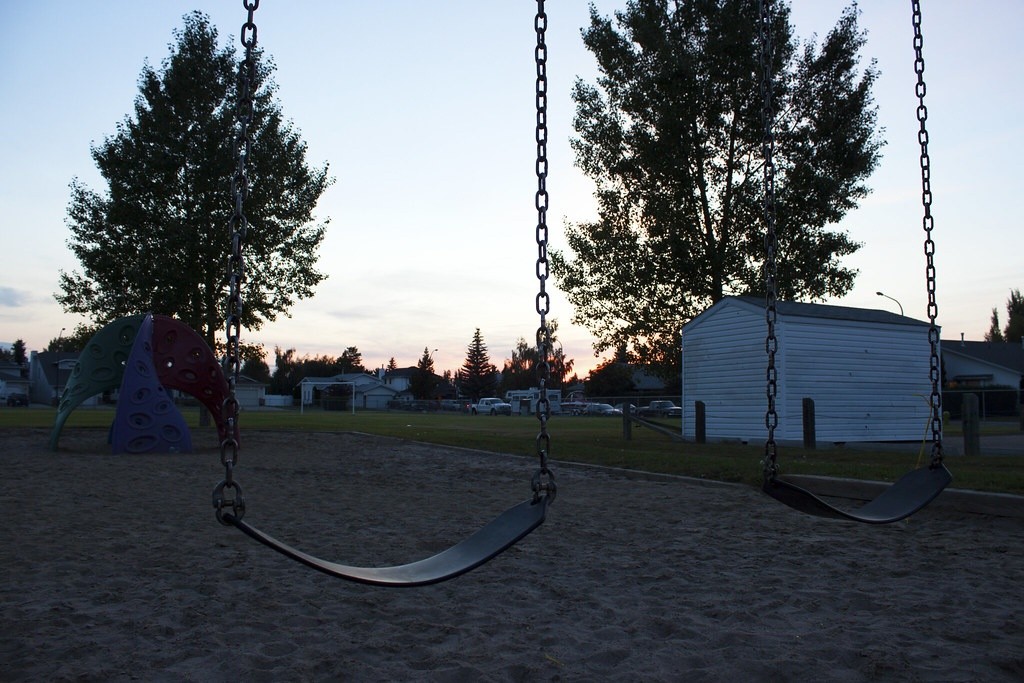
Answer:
[746,2,950,527]
[213,0,556,587]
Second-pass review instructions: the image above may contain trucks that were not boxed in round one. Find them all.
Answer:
[504,390,561,415]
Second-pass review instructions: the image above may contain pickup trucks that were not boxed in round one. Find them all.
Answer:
[468,398,511,417]
[637,401,682,419]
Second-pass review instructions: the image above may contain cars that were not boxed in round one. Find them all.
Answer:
[615,403,637,415]
[561,402,587,415]
[583,404,622,417]
